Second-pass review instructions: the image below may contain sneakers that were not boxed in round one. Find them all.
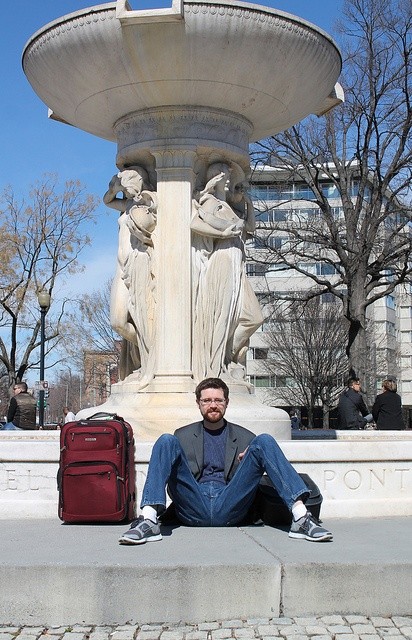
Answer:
[119,514,163,545]
[288,510,333,542]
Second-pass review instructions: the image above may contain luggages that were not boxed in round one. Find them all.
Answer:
[56,411,137,524]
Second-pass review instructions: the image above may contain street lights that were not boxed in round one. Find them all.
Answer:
[38,288,50,429]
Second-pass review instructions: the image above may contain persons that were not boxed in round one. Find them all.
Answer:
[0,382,37,430]
[372,380,403,430]
[289,411,302,429]
[63,407,76,424]
[190,159,263,394]
[338,377,374,430]
[294,409,307,430]
[103,166,157,390]
[118,378,333,545]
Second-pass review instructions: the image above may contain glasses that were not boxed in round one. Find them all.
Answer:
[199,398,228,404]
[14,387,20,390]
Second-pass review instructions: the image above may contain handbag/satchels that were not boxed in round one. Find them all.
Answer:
[259,471,323,527]
